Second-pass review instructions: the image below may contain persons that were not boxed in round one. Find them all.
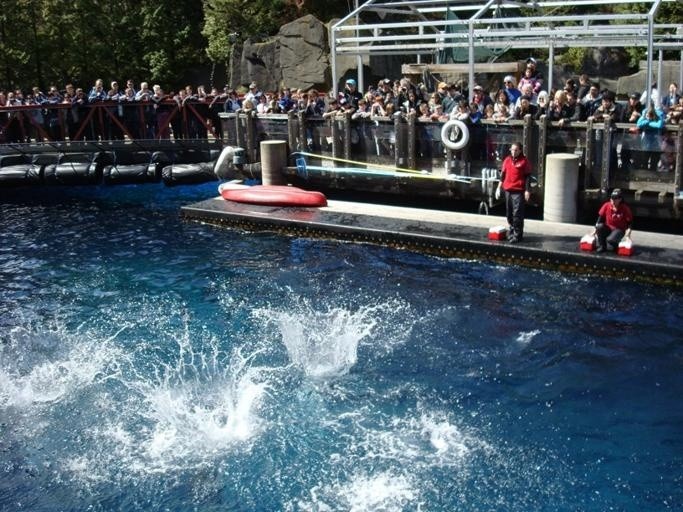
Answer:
[0,56,682,177]
[590,187,633,254]
[494,141,532,243]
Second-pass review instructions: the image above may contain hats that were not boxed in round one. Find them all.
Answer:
[383,79,391,83]
[504,76,515,87]
[438,82,447,89]
[526,57,536,63]
[249,84,256,88]
[631,92,640,99]
[611,189,621,196]
[329,98,336,104]
[473,85,482,91]
[346,79,356,86]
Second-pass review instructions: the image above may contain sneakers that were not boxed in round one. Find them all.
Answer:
[508,234,518,243]
[597,245,604,252]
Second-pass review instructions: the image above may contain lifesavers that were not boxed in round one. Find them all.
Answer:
[441,120,469,150]
